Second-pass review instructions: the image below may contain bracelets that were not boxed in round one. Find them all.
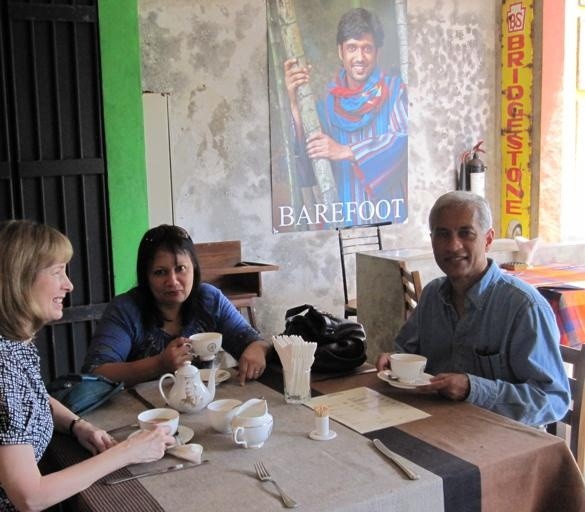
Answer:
[70,416,87,435]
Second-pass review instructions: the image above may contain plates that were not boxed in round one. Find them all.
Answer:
[127,425,195,450]
[309,431,336,441]
[199,369,230,382]
[377,369,434,389]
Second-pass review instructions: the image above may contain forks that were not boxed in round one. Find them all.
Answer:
[254,462,297,507]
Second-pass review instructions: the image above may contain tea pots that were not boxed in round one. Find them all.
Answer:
[159,360,217,412]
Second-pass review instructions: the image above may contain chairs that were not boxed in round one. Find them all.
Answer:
[545,343,585,480]
[193,240,278,334]
[335,220,395,320]
[399,262,422,323]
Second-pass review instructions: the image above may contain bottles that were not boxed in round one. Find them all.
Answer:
[316,416,329,435]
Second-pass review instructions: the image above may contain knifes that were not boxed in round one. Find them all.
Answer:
[104,459,209,485]
[373,439,418,480]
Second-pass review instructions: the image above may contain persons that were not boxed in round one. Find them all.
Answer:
[80,223,271,386]
[375,189,572,428]
[284,8,409,231]
[0,218,177,512]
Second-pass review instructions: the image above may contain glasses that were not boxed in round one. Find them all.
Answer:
[145,225,190,243]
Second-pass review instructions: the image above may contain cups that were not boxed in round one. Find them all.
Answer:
[208,398,244,433]
[138,407,179,435]
[390,353,427,382]
[284,369,312,404]
[183,333,222,361]
[231,417,273,447]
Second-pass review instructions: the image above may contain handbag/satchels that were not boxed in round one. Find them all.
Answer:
[271,304,367,373]
[46,371,125,416]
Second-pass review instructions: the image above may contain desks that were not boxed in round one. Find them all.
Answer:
[356,237,585,364]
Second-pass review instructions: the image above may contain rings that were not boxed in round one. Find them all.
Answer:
[254,370,260,374]
